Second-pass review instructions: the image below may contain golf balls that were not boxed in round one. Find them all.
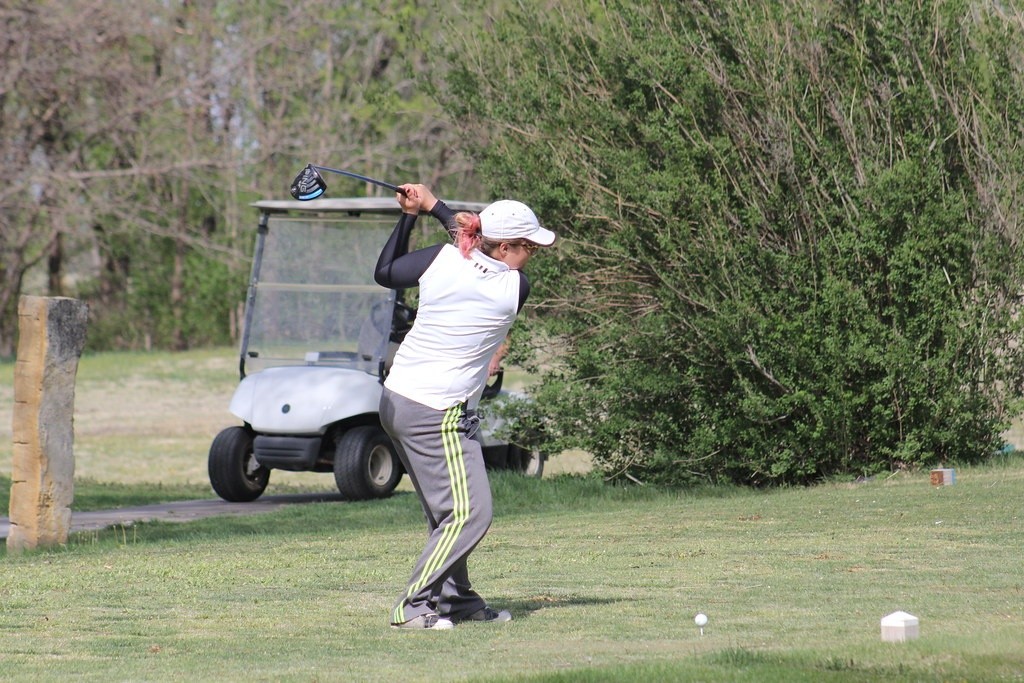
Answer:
[695,614,708,626]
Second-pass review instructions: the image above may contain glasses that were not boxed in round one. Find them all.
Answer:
[487,242,539,255]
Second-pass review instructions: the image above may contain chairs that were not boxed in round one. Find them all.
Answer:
[357,318,409,369]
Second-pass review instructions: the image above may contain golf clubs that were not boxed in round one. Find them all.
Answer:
[289,162,407,201]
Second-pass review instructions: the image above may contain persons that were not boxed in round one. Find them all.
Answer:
[374,183,556,630]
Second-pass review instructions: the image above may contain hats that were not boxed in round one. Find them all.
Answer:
[479,200,556,247]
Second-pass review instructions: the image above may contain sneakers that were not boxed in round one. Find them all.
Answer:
[389,610,454,630]
[434,604,512,622]
